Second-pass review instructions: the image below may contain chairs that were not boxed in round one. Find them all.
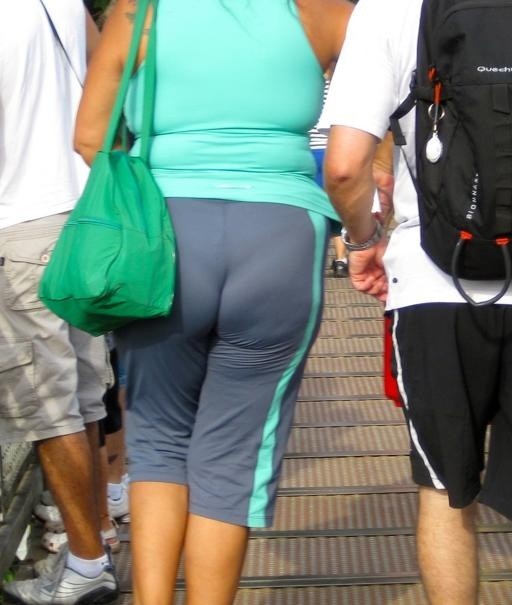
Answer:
[1,474,128,602]
[332,254,353,278]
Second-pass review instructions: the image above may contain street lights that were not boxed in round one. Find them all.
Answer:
[37,151,175,336]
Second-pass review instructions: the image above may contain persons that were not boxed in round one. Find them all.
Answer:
[313,0,511,605]
[75,0,360,605]
[1,0,124,605]
[308,59,351,277]
[33,325,137,559]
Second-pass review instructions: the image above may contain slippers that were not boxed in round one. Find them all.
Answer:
[390,1,509,278]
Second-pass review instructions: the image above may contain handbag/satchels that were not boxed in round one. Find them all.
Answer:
[340,216,385,252]
